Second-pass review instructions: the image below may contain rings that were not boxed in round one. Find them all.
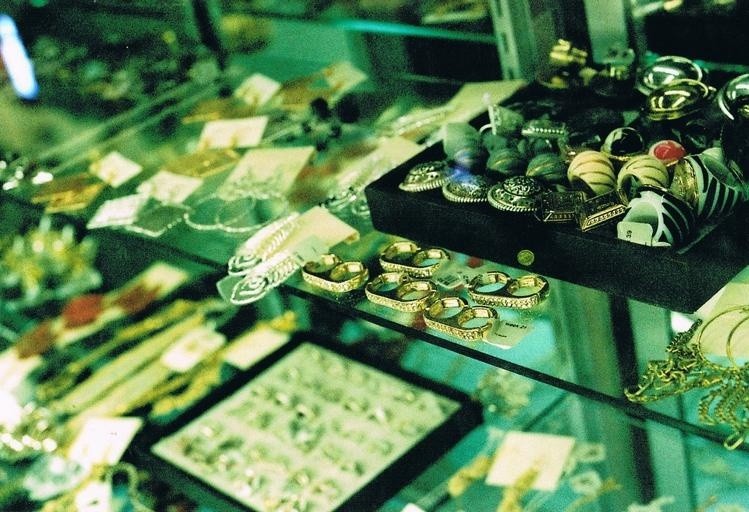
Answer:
[175,347,440,512]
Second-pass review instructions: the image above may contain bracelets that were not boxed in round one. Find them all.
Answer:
[626,303,748,450]
[180,190,290,234]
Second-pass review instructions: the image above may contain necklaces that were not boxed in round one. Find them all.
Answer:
[1,66,235,190]
